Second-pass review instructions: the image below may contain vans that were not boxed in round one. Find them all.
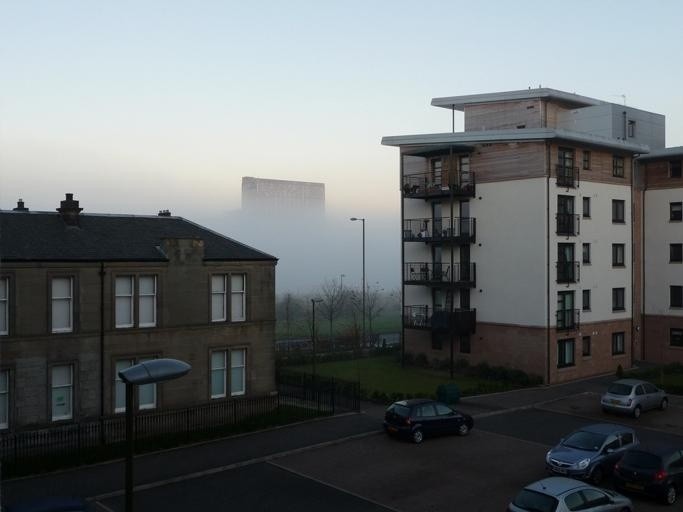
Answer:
[614,439,683,504]
[384,398,474,444]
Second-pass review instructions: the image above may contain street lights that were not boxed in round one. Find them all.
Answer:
[340,218,366,347]
[118,358,192,511]
[311,297,323,400]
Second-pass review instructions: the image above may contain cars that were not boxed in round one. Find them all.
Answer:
[600,379,669,419]
[545,422,640,485]
[508,477,634,511]
